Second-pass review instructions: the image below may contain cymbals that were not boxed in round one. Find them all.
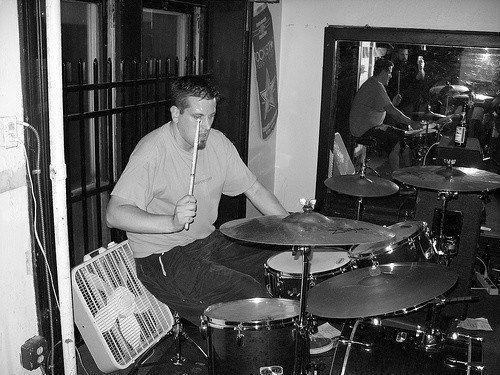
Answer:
[428,84,470,95]
[451,93,494,101]
[392,164,500,193]
[324,174,400,199]
[410,112,448,121]
[305,260,459,320]
[217,209,397,249]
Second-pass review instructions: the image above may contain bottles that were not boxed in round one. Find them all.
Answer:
[455,101,469,147]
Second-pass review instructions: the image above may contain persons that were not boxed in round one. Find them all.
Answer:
[105,75,334,355]
[384,48,426,120]
[349,58,422,194]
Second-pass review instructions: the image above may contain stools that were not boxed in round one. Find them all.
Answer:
[354,139,381,177]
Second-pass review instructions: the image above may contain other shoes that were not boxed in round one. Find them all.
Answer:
[398,183,416,195]
[309,336,333,354]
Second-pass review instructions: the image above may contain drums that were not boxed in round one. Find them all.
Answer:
[404,129,438,150]
[199,297,311,375]
[262,246,353,301]
[324,320,486,375]
[349,218,441,268]
[437,113,464,143]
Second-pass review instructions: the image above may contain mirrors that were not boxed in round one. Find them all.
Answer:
[314,26,500,257]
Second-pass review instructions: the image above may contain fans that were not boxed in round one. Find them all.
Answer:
[71,239,175,374]
[327,133,355,183]
